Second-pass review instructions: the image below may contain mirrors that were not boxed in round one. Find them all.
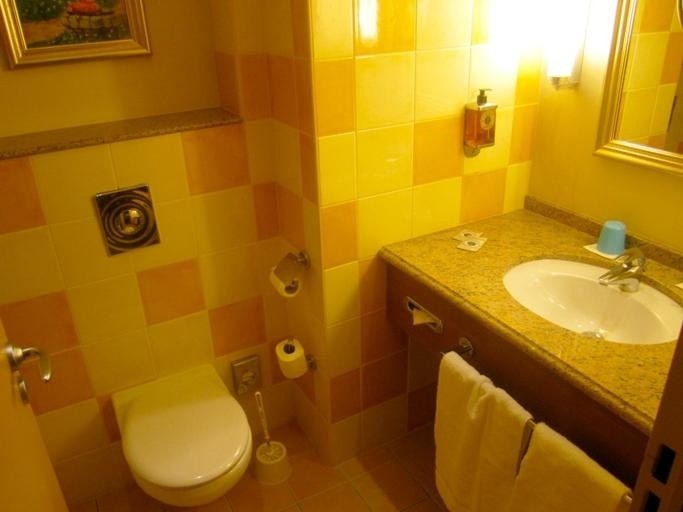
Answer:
[590,0,681,176]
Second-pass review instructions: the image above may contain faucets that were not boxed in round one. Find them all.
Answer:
[599,248,647,293]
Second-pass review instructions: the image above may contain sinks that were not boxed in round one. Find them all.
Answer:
[502,254,683,345]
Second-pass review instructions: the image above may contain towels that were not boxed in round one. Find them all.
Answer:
[433,348,633,511]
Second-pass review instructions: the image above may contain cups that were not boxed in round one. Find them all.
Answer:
[596,220,625,255]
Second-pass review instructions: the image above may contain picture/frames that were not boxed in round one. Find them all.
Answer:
[0,1,153,71]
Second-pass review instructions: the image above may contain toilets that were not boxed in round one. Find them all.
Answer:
[111,364,253,508]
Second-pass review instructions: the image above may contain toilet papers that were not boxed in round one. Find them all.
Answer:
[276,338,309,379]
[271,266,302,297]
